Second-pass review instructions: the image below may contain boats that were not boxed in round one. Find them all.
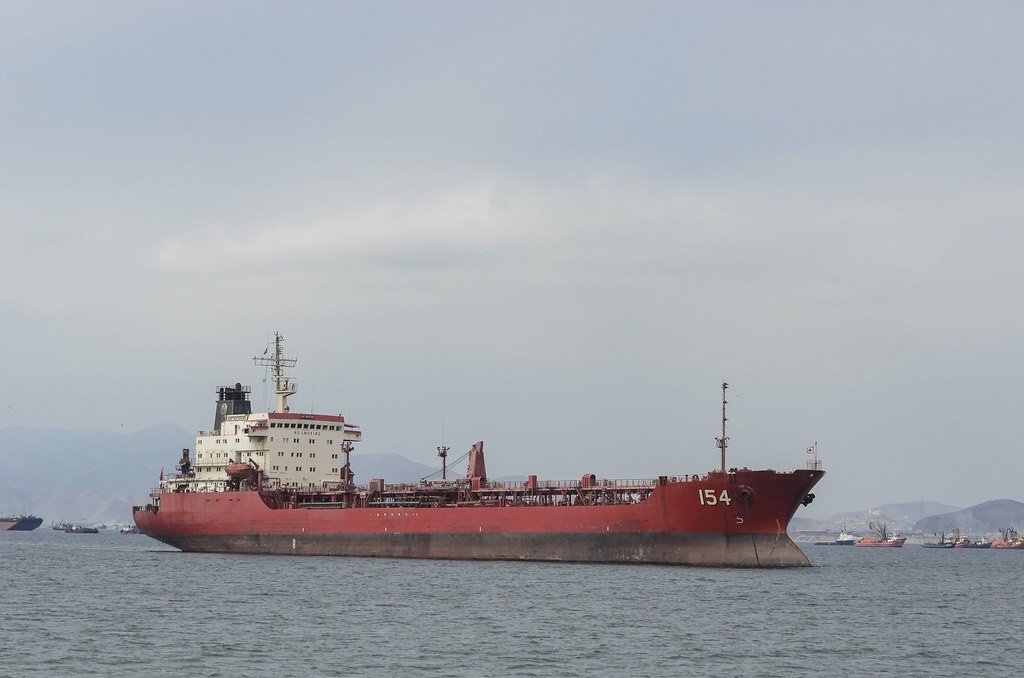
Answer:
[955,536,992,548]
[65,525,99,534]
[815,529,861,546]
[0,514,43,531]
[921,530,955,548]
[853,520,907,548]
[120,525,136,535]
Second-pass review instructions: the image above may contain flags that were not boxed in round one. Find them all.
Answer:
[158,468,165,481]
[806,447,815,454]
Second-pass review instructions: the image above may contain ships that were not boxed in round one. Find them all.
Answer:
[132,331,826,568]
